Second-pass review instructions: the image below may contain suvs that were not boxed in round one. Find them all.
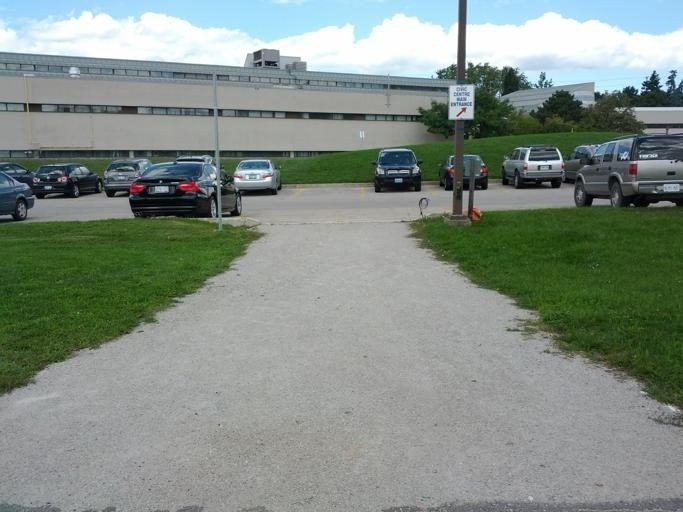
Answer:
[502,143,565,188]
[574,132,683,209]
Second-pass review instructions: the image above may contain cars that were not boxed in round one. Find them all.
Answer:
[129,161,242,218]
[562,144,601,182]
[0,162,36,221]
[103,158,153,197]
[438,154,488,191]
[32,162,103,199]
[233,159,282,195]
[370,148,423,192]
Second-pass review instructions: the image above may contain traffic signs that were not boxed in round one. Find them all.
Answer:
[448,84,476,121]
